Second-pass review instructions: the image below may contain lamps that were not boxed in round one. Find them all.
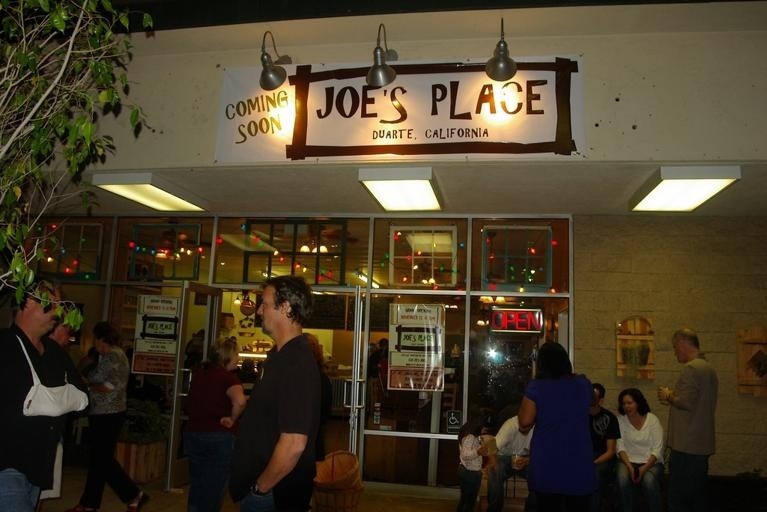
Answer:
[90,172,214,212]
[353,167,443,213]
[259,30,293,92]
[628,167,740,215]
[484,16,517,81]
[298,238,329,254]
[367,23,398,88]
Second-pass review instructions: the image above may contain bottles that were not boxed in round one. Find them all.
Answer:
[373,402,382,424]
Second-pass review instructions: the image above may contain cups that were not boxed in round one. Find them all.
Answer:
[512,448,521,470]
[660,386,671,405]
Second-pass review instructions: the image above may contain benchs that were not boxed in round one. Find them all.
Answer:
[609,473,767,512]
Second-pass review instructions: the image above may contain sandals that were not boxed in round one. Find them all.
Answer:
[65,503,98,512]
[127,490,150,512]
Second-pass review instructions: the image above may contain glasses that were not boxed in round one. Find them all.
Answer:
[225,336,237,352]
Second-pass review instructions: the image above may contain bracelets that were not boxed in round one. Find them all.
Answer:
[667,393,673,401]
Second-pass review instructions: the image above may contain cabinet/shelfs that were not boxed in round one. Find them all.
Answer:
[108,261,163,351]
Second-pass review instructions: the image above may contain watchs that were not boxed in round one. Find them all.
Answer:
[250,483,267,497]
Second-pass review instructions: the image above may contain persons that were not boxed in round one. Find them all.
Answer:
[45,315,72,348]
[589,381,622,487]
[226,274,322,511]
[368,338,387,368]
[487,415,536,512]
[454,417,488,511]
[184,337,247,512]
[184,328,205,369]
[656,327,719,512]
[0,278,90,511]
[518,341,598,512]
[301,333,333,482]
[64,320,150,511]
[613,387,666,512]
